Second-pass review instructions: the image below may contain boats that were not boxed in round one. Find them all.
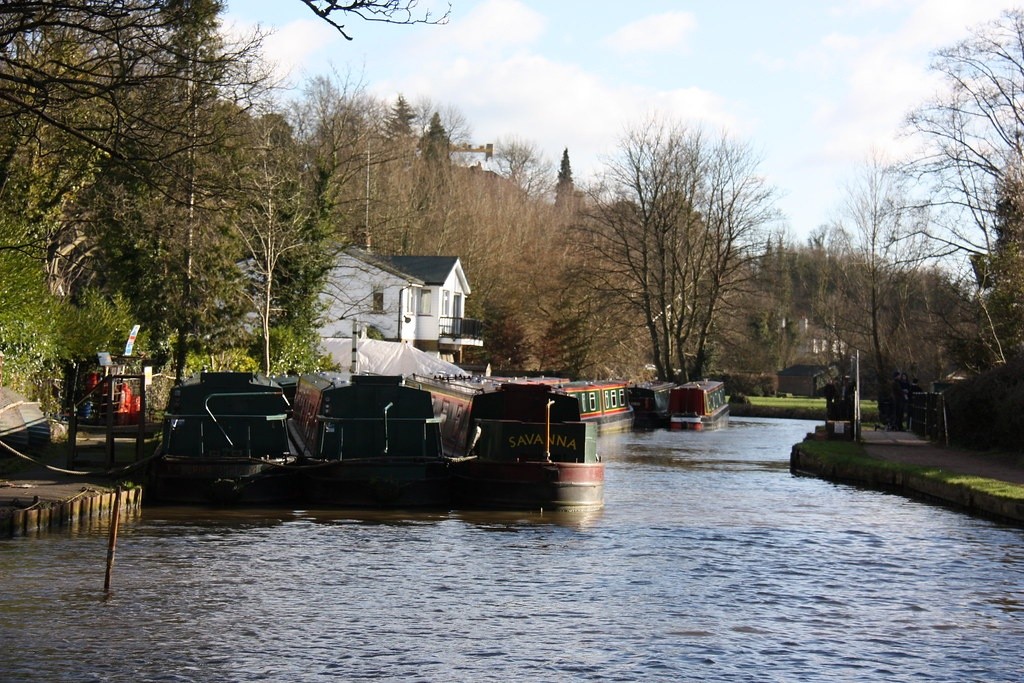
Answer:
[287,375,454,521]
[403,375,499,458]
[503,375,571,389]
[628,381,673,429]
[138,372,299,519]
[670,378,731,431]
[547,380,636,436]
[0,385,54,479]
[448,383,605,528]
